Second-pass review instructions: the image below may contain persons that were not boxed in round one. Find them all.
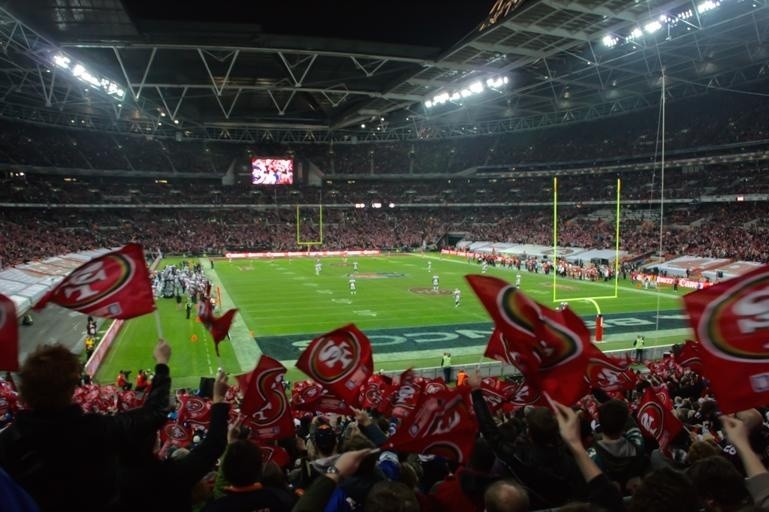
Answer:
[317,260,321,272]
[145,256,219,321]
[1,121,416,269]
[594,310,604,341]
[0,333,766,510]
[314,261,319,276]
[351,258,360,274]
[453,287,462,311]
[347,276,357,296]
[418,159,768,298]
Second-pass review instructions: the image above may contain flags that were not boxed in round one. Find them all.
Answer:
[0,290,22,375]
[26,239,158,323]
[190,280,239,358]
[292,320,376,409]
[461,271,633,408]
[680,263,768,416]
